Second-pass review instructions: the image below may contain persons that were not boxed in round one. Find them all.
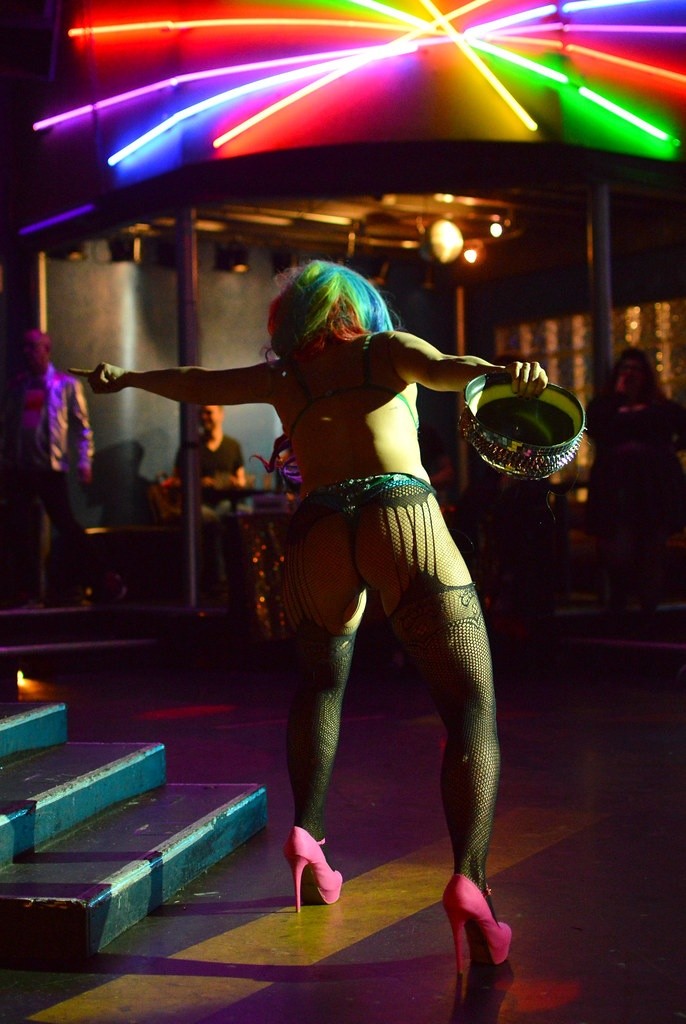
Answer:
[441,462,550,636]
[172,402,254,522]
[2,330,126,609]
[418,421,458,506]
[581,349,683,635]
[70,263,548,968]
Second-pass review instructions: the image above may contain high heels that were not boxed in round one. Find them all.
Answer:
[435,871,511,974]
[281,825,343,915]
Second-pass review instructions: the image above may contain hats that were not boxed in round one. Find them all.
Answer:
[453,362,586,482]
